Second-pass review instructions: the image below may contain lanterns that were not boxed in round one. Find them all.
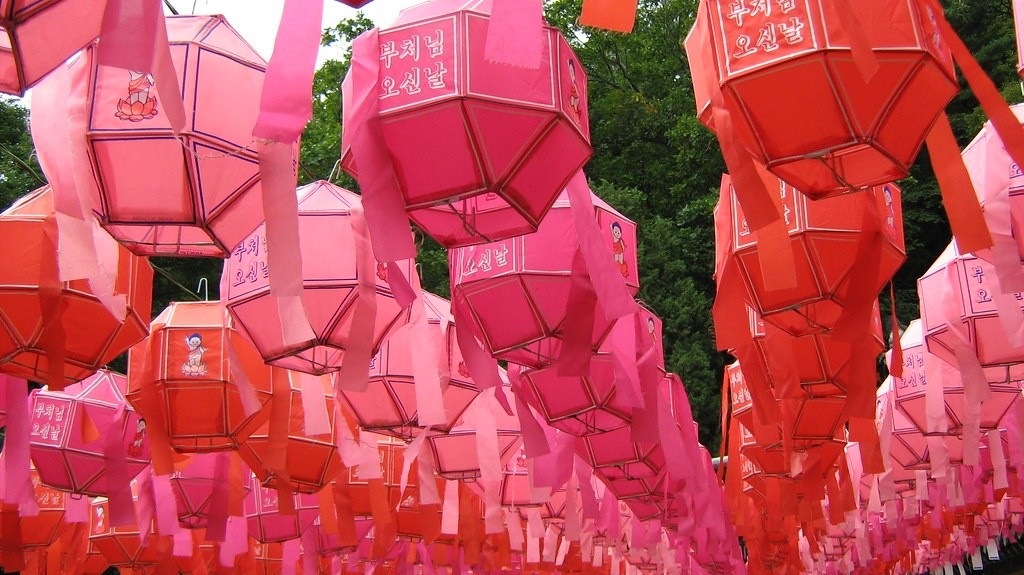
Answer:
[1,0,1024,575]
